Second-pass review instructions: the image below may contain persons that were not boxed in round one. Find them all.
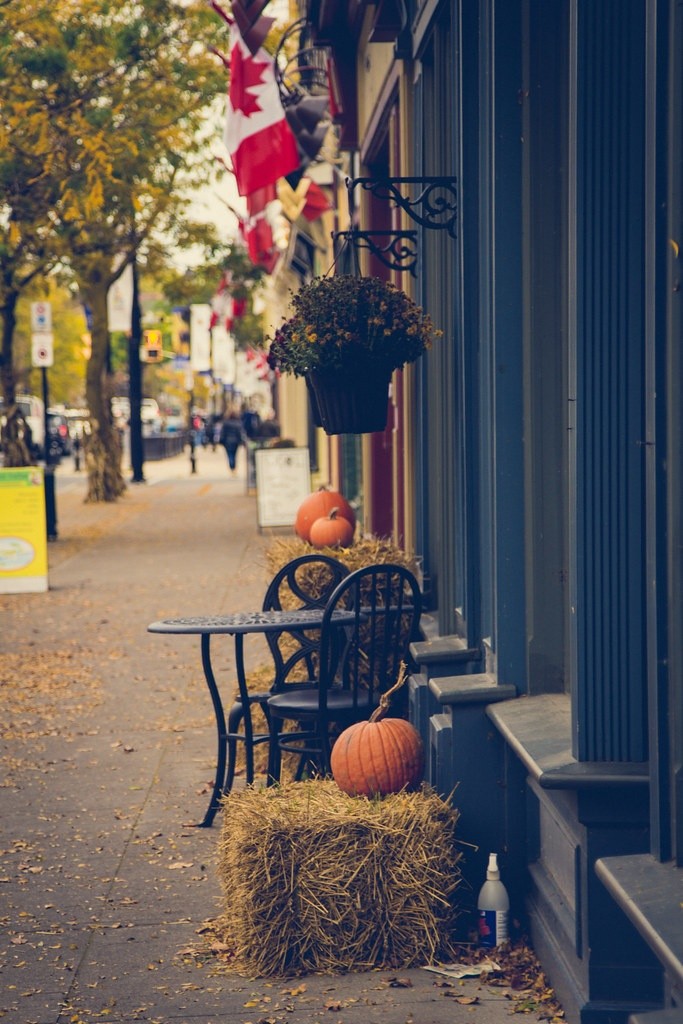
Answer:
[219,404,253,470]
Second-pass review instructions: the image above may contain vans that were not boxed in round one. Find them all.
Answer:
[0,395,46,460]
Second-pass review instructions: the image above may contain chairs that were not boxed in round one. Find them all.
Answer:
[266,564,423,781]
[217,554,354,807]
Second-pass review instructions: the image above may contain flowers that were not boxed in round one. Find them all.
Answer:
[263,316,299,374]
[264,275,444,377]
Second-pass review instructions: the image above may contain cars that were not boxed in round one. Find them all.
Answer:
[41,398,161,459]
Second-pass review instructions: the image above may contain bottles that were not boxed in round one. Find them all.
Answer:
[477,853,510,953]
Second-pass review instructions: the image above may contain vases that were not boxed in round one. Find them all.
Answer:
[304,374,324,428]
[309,361,392,436]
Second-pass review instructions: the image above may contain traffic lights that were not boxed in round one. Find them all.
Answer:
[145,330,162,364]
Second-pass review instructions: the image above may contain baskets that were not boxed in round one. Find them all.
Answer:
[304,363,393,436]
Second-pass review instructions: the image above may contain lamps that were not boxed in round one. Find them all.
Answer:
[272,15,334,162]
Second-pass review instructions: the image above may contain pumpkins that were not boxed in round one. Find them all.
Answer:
[309,507,354,551]
[331,703,423,800]
[295,485,355,545]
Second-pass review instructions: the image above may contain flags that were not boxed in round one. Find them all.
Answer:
[208,25,330,380]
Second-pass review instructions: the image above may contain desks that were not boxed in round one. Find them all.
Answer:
[146,610,369,827]
